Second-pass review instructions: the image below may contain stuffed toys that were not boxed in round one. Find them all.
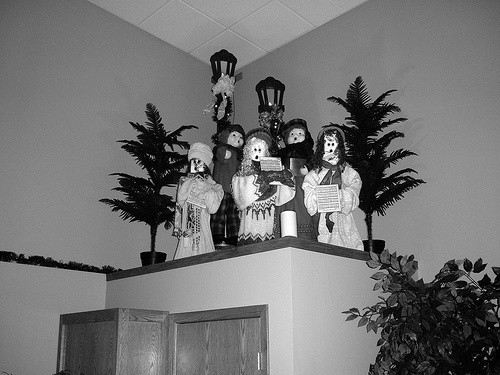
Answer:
[172,142,224,260]
[210,125,246,247]
[230,128,296,246]
[273,118,320,242]
[301,126,364,251]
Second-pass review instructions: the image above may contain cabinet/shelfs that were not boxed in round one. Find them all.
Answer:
[56,304,268,375]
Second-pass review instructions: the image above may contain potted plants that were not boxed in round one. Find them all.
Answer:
[321,76,426,255]
[98,101,199,267]
